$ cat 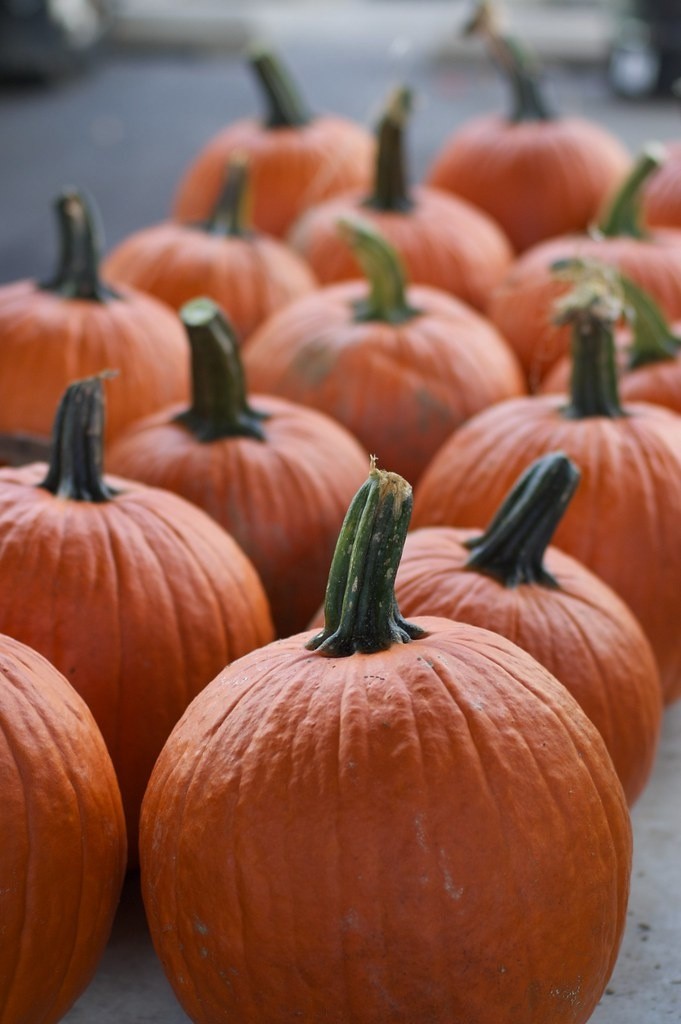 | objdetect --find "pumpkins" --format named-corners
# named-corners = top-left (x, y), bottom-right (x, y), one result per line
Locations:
top-left (0, 0), bottom-right (681, 1024)
